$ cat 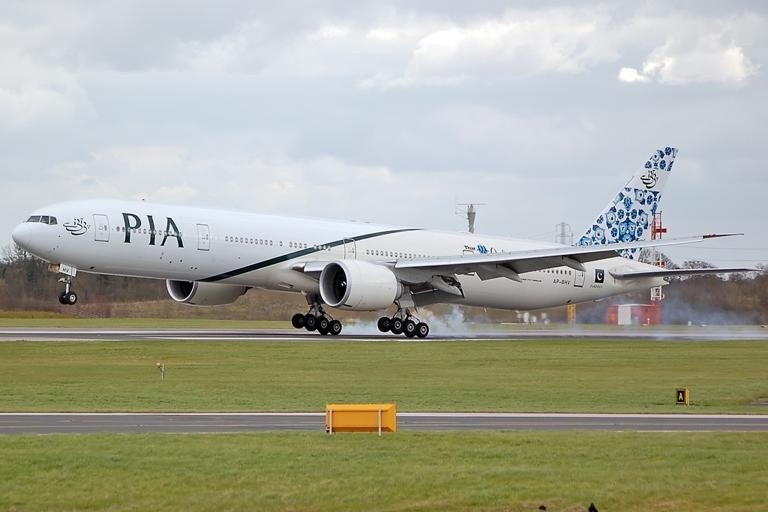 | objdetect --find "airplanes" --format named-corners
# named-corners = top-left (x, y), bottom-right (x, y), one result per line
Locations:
top-left (9, 146), bottom-right (762, 339)
